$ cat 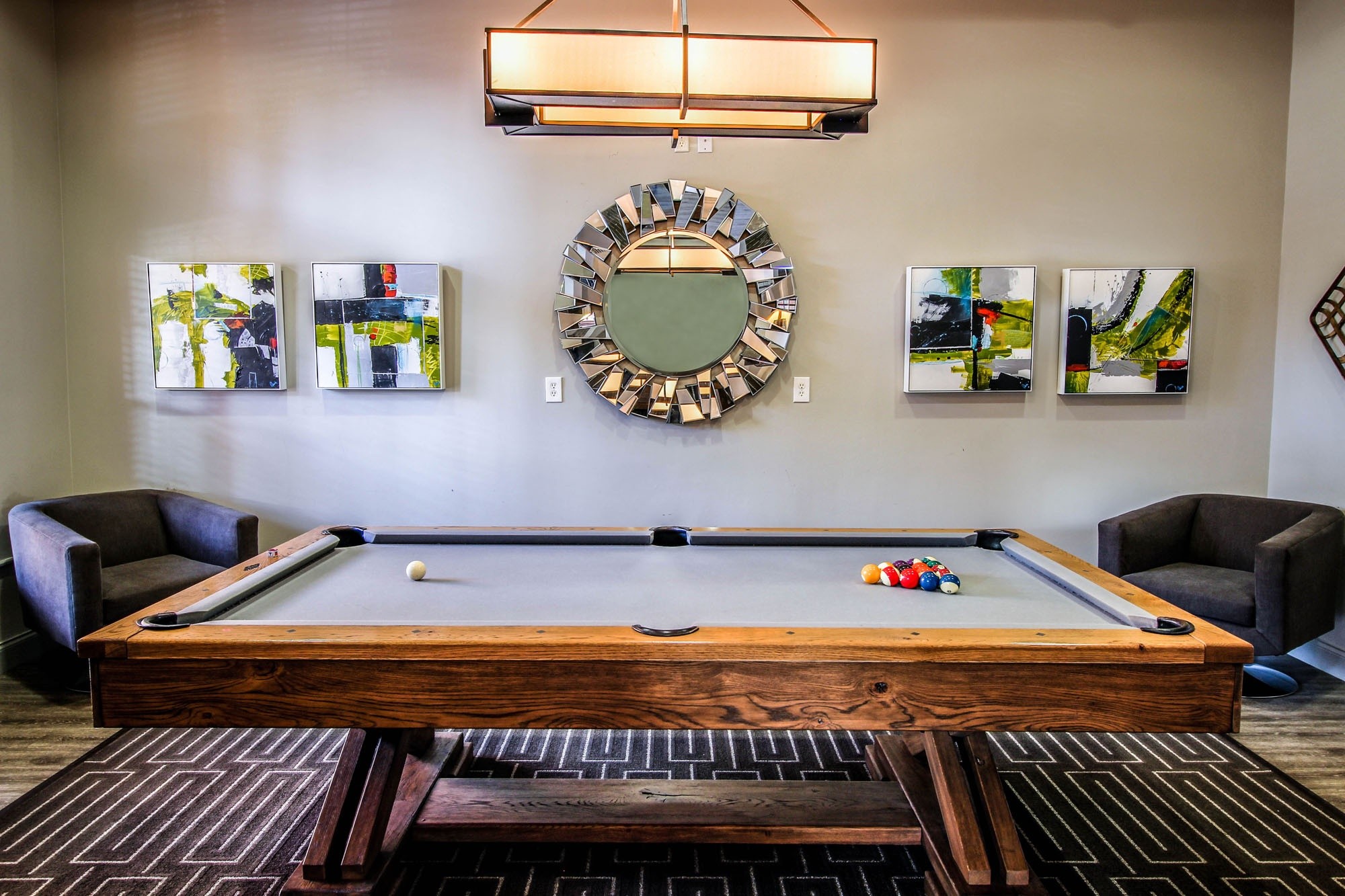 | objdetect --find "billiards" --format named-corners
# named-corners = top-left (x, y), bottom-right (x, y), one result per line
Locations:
top-left (860, 557), bottom-right (960, 594)
top-left (406, 560), bottom-right (426, 580)
